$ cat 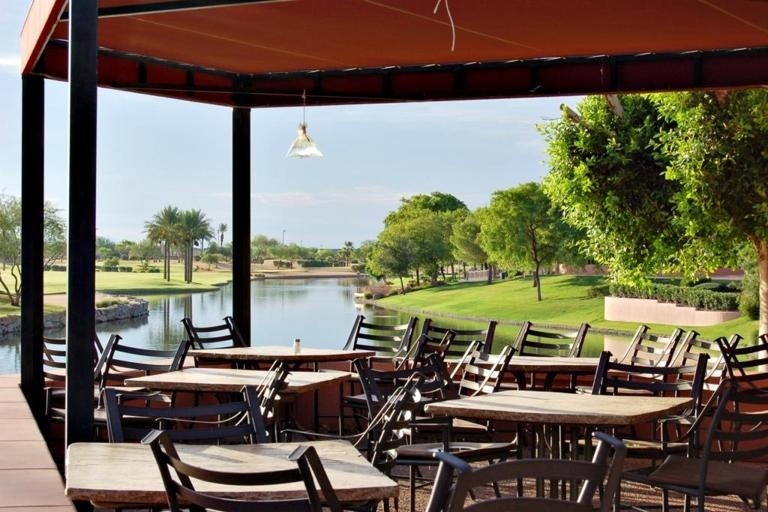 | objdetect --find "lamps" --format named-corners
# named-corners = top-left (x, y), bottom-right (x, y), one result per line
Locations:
top-left (286, 91), bottom-right (323, 162)
